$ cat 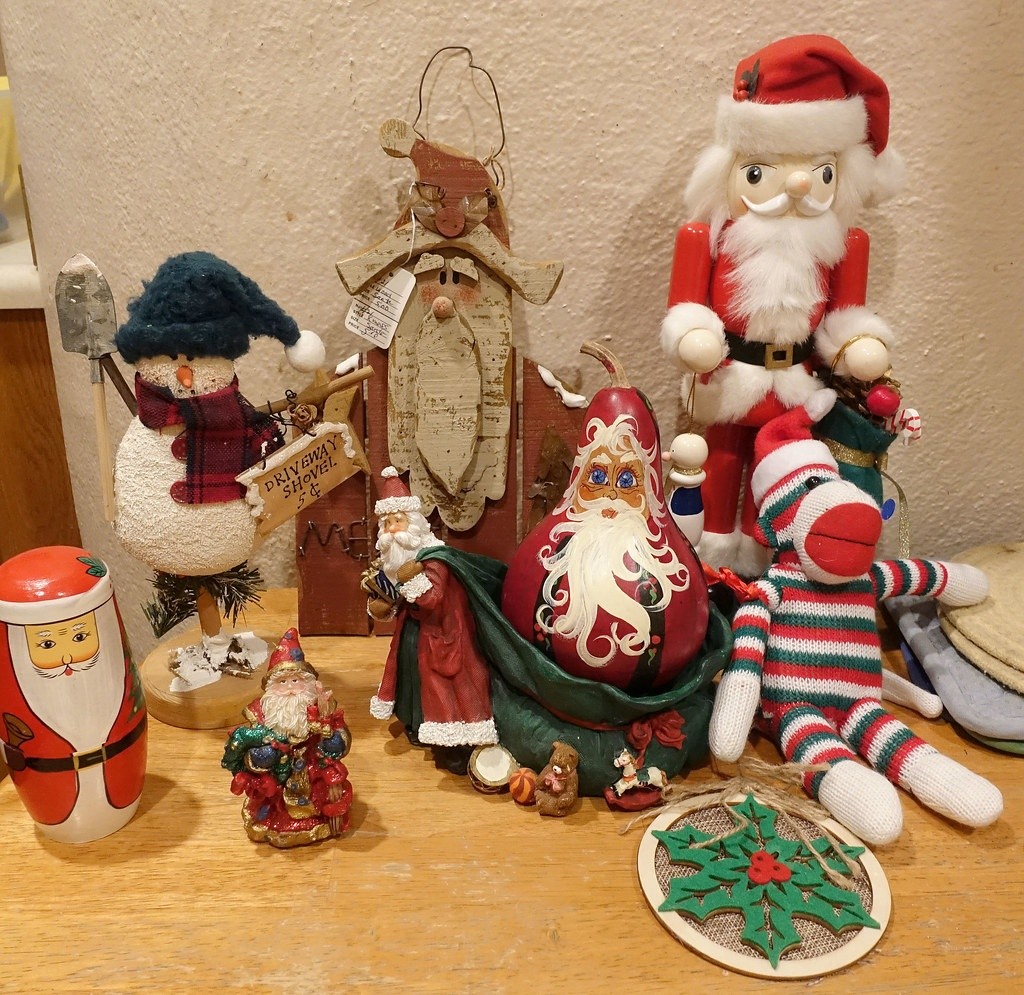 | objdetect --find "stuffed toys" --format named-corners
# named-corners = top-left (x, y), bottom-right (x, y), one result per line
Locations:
top-left (656, 35), bottom-right (906, 586)
top-left (706, 387), bottom-right (1004, 846)
